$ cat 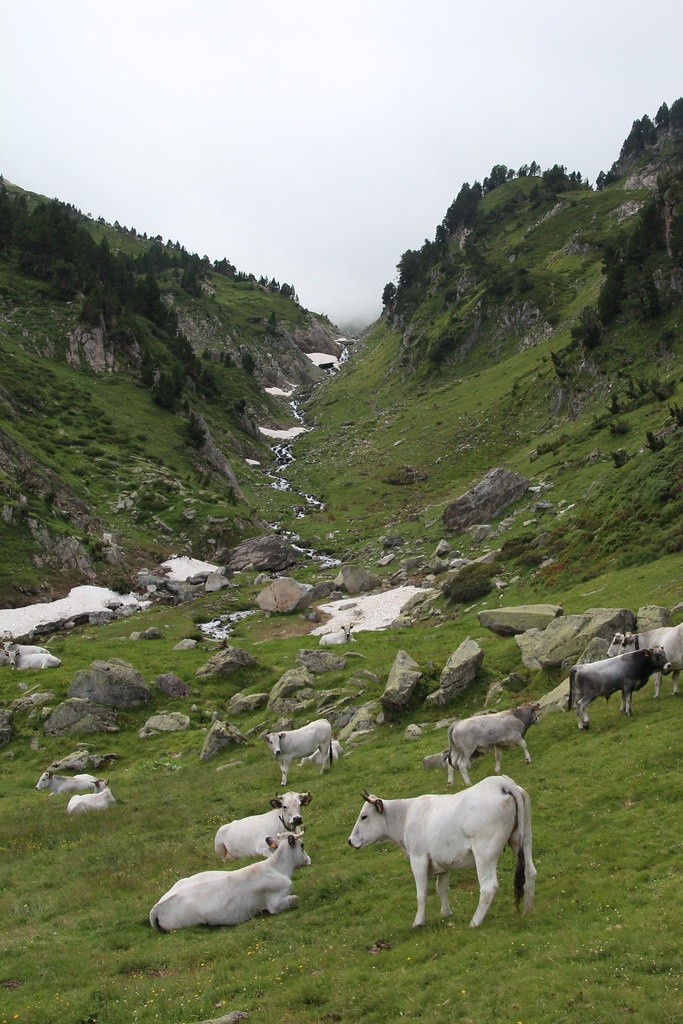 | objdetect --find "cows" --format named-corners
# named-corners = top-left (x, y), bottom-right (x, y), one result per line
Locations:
top-left (265, 719), bottom-right (332, 785)
top-left (1, 639), bottom-right (51, 654)
top-left (297, 740), bottom-right (343, 767)
top-left (319, 625), bottom-right (357, 645)
top-left (36, 772), bottom-right (99, 797)
top-left (607, 623), bottom-right (683, 698)
top-left (150, 827), bottom-right (311, 929)
top-left (347, 774), bottom-right (537, 929)
top-left (447, 703), bottom-right (540, 784)
top-left (66, 780), bottom-right (116, 816)
top-left (566, 650), bottom-right (671, 730)
top-left (4, 649), bottom-right (62, 669)
top-left (214, 789), bottom-right (313, 862)
